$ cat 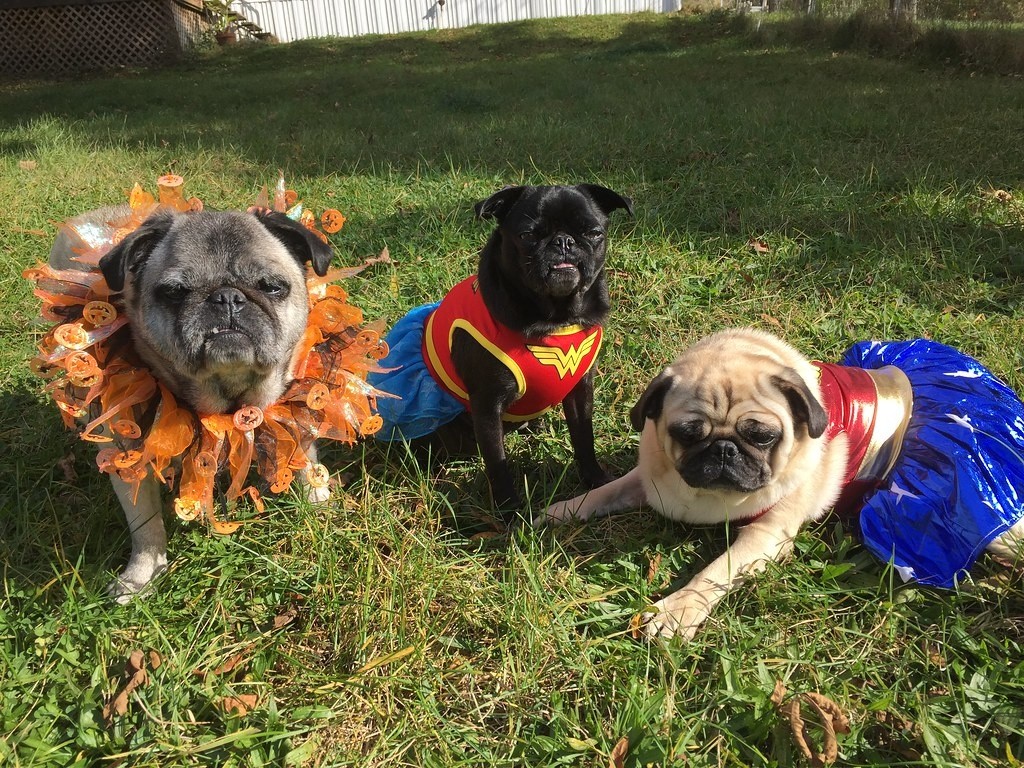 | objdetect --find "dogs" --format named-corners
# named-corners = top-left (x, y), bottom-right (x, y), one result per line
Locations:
top-left (46, 181), bottom-right (1024, 649)
